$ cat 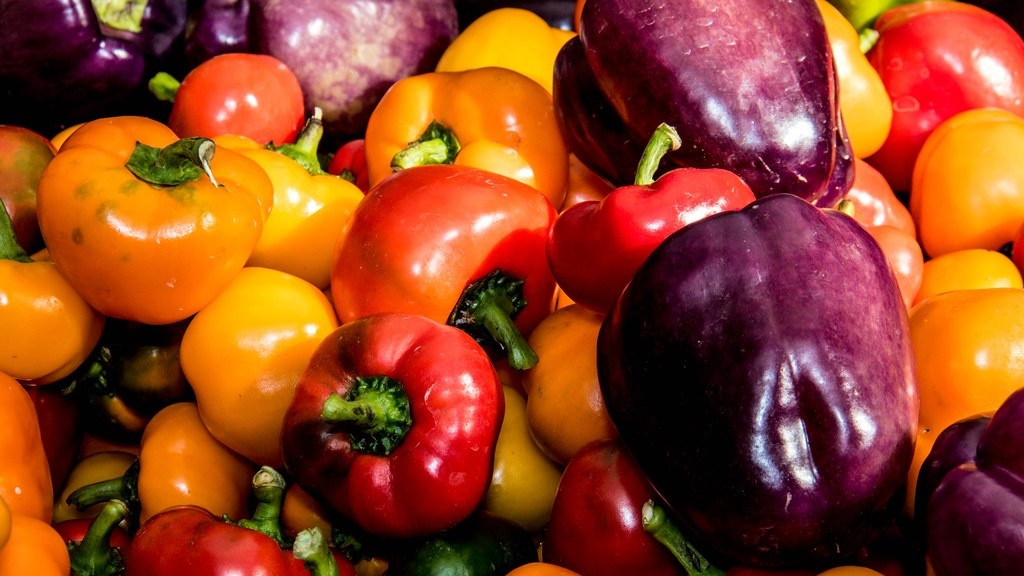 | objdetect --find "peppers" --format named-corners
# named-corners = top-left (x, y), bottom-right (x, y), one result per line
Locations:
top-left (0, 0), bottom-right (1024, 576)
